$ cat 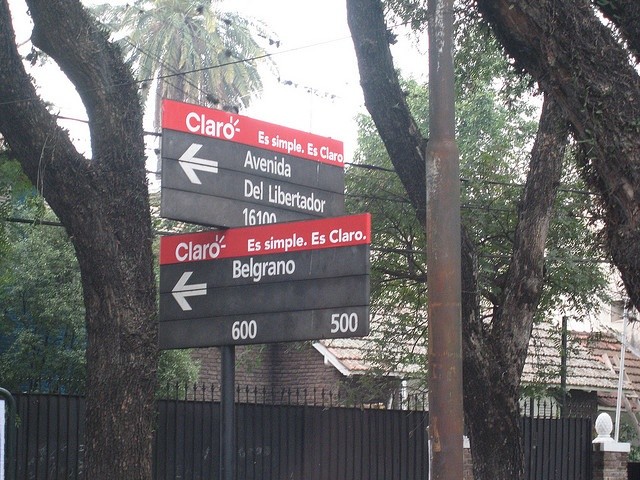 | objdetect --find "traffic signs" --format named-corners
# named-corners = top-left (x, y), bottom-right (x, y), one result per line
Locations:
top-left (161, 99), bottom-right (346, 228)
top-left (158, 212), bottom-right (371, 351)
